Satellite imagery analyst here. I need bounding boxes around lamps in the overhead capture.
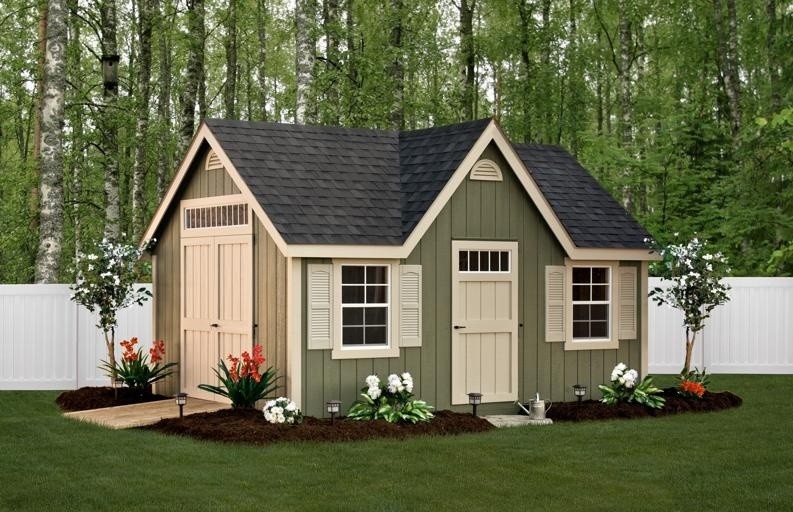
[467,384,586,415]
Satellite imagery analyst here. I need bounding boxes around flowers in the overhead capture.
[258,390,305,426]
[94,335,177,392]
[598,363,712,411]
[347,369,434,425]
[196,344,288,409]
